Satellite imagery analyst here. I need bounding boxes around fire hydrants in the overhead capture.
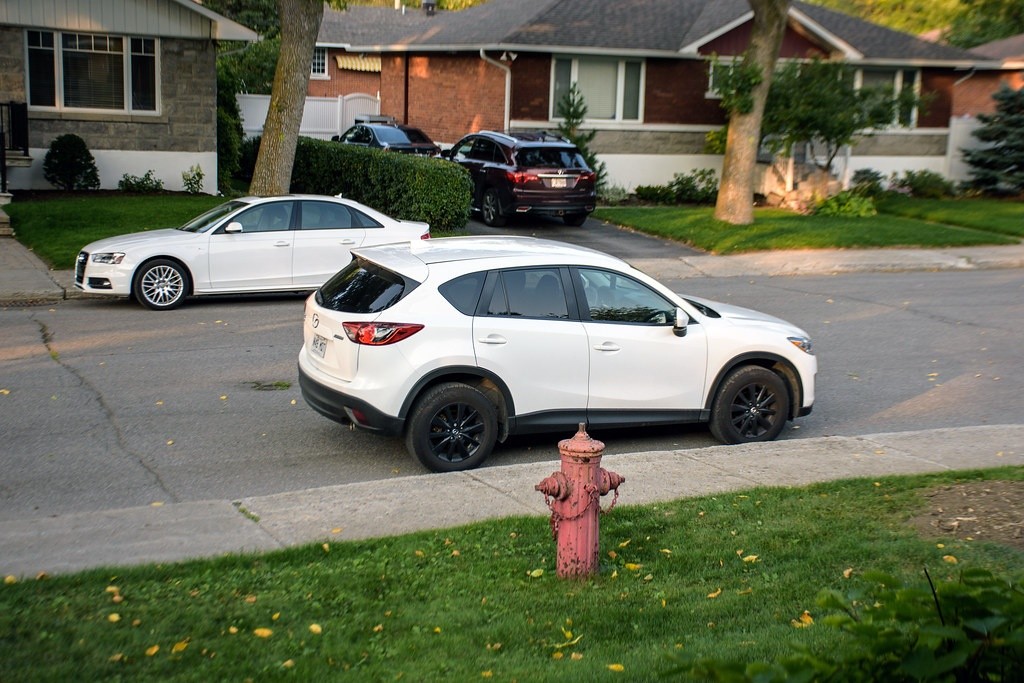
[533,420,628,585]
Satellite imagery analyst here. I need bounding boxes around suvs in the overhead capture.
[431,128,601,227]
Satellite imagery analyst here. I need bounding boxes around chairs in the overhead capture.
[264,206,288,229]
[535,275,563,316]
[504,271,534,317]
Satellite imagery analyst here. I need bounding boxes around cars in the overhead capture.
[297,234,820,476]
[329,123,441,161]
[72,193,432,312]
